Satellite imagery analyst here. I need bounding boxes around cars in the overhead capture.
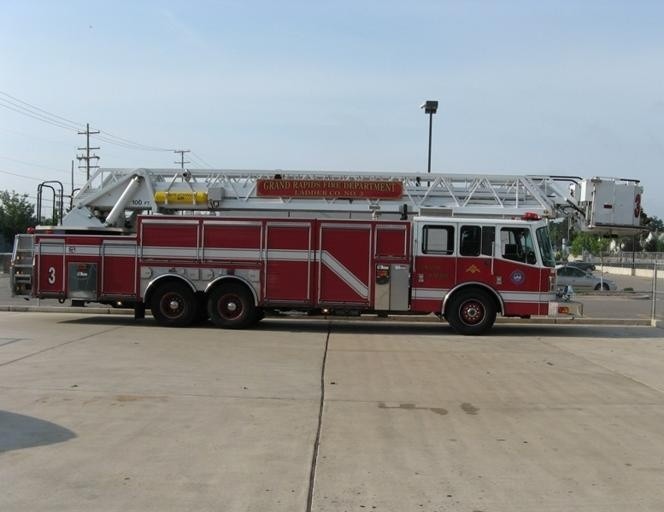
[554,260,616,291]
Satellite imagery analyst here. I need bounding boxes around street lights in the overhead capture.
[423,99,438,185]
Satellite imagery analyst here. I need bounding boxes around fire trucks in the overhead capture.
[10,168,651,336]
